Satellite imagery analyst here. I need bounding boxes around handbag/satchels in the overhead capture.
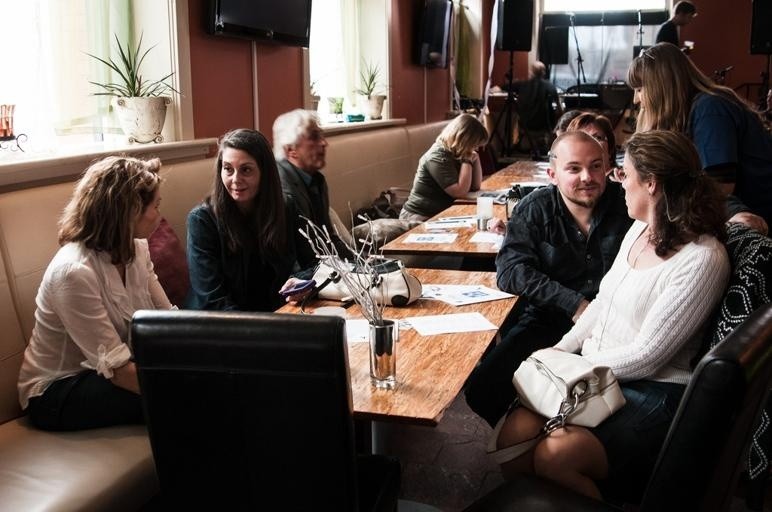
[301,256,422,317]
[484,346,626,464]
[506,184,547,221]
[356,190,398,222]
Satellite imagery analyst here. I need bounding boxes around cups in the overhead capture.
[476,197,494,230]
[507,198,520,220]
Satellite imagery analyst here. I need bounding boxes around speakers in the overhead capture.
[496,0,533,51]
[538,11,570,64]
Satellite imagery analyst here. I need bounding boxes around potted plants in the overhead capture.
[352,55,387,120]
[311,80,320,110]
[82,29,186,146]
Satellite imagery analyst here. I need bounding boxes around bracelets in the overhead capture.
[461,158,473,168]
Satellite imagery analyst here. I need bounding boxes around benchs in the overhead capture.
[0,123,451,511]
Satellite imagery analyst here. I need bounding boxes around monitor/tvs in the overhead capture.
[411,0,453,69]
[211,0,312,49]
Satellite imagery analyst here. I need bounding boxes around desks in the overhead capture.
[274,266,519,455]
[379,162,551,259]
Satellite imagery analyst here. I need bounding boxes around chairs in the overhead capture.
[131,309,402,512]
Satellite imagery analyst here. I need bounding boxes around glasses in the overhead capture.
[639,48,655,62]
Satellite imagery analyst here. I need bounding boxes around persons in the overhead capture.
[553,109,582,138]
[760,88,772,120]
[398,112,495,273]
[513,60,564,156]
[607,42,771,242]
[493,128,734,503]
[484,111,622,238]
[185,127,314,317]
[655,0,698,58]
[269,107,353,266]
[463,128,769,429]
[15,155,179,432]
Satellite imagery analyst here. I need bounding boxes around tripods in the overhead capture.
[483,51,542,160]
